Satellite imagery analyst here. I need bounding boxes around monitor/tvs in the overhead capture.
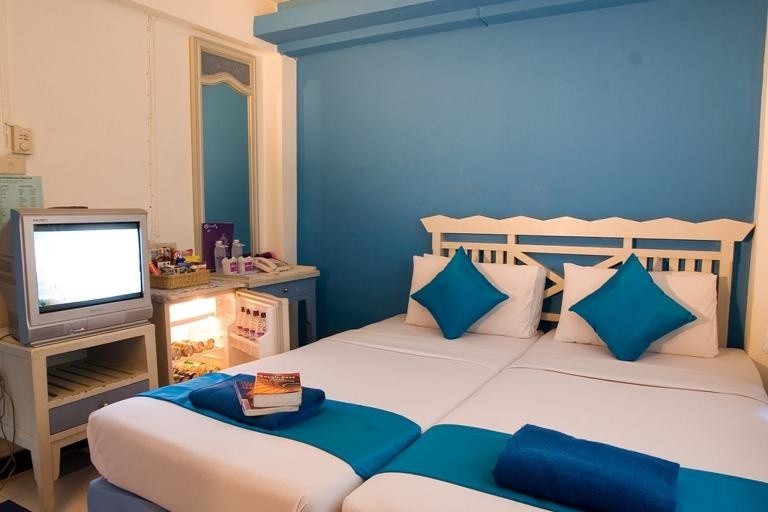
[0,206,154,347]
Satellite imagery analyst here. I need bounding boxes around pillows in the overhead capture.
[412,245,509,340]
[568,252,698,362]
[553,263,721,359]
[406,253,546,338]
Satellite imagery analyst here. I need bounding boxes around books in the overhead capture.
[234,380,300,416]
[254,371,303,406]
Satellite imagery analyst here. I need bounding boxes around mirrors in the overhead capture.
[190,37,260,255]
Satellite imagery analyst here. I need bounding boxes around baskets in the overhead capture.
[150,269,211,289]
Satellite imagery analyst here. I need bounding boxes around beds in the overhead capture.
[86,214,767,512]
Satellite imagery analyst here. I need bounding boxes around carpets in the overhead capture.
[0,500,32,512]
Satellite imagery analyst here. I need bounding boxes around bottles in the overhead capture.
[232,239,243,258]
[214,240,226,274]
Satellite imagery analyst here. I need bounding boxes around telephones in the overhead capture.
[254,257,290,273]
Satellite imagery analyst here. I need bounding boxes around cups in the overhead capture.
[238,256,254,274]
[222,257,237,275]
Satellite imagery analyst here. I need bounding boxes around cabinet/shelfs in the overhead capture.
[0,324,159,512]
[247,276,316,349]
